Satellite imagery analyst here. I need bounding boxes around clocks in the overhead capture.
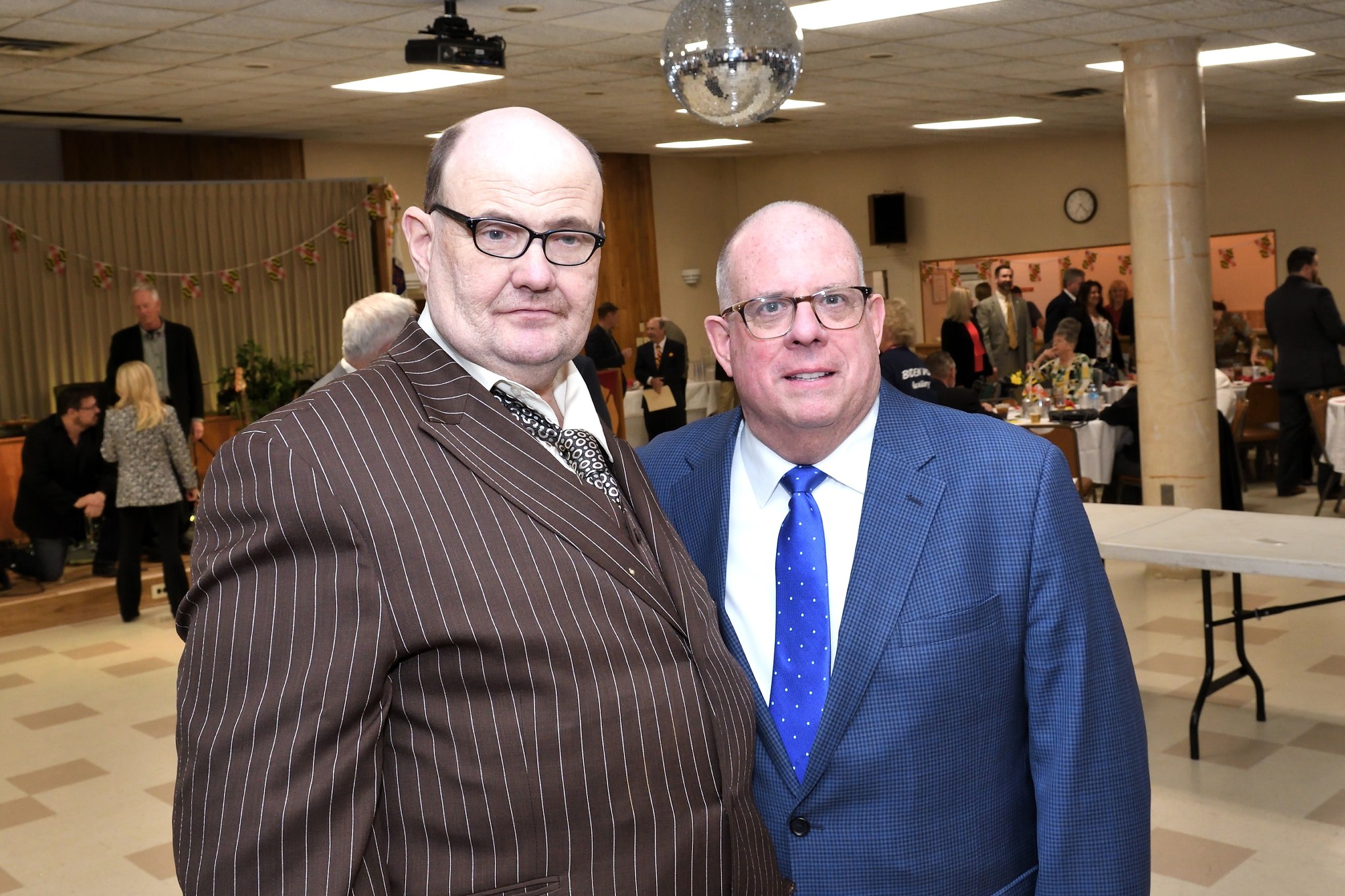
[1063,187,1098,224]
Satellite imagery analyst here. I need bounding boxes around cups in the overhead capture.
[1079,396,1092,409]
[995,403,1009,420]
[1053,386,1065,407]
[1029,406,1042,423]
[1233,363,1243,381]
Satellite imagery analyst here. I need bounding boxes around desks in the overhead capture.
[1100,379,1136,405]
[1230,378,1252,401]
[989,404,1129,500]
[1324,393,1345,511]
[1082,501,1192,570]
[622,379,722,450]
[1099,506,1345,762]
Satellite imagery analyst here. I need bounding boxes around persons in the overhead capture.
[1263,246,1345,500]
[101,362,197,623]
[585,302,627,400]
[1213,310,1260,366]
[878,298,938,404]
[940,265,1141,505]
[925,351,985,414]
[305,292,417,394]
[636,202,1152,896]
[572,355,612,431]
[715,359,740,414]
[0,387,116,590]
[105,289,205,563]
[172,105,794,896]
[633,317,687,443]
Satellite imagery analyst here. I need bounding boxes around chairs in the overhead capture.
[602,345,1345,517]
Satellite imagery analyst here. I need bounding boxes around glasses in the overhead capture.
[73,402,101,412]
[429,204,606,265]
[720,284,873,339]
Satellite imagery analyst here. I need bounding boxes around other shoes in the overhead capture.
[1278,485,1306,496]
[119,609,140,621]
[146,551,163,563]
[1301,476,1319,486]
[93,566,117,578]
[180,533,192,554]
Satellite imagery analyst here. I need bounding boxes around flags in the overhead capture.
[180,184,398,299]
[920,252,1132,287]
[1218,248,1236,269]
[1254,236,1276,258]
[6,224,156,289]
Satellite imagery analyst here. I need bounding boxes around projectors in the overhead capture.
[404,38,507,71]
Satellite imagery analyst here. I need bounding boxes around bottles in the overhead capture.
[1088,384,1097,409]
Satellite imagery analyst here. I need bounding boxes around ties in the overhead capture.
[488,386,624,515]
[767,466,831,788]
[656,345,662,368]
[1005,296,1019,349]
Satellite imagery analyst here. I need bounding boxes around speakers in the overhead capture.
[867,191,909,245]
